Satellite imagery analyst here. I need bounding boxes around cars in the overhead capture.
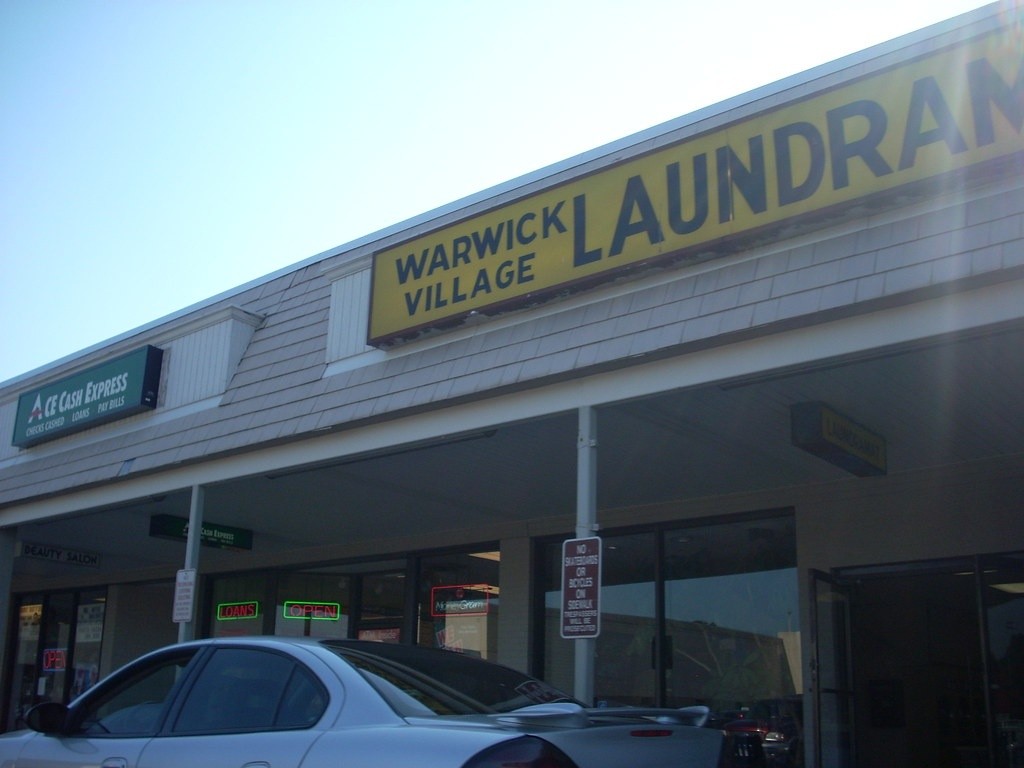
[0,636,728,768]
[709,698,798,768]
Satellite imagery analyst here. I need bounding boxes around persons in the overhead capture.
[69,664,99,704]
